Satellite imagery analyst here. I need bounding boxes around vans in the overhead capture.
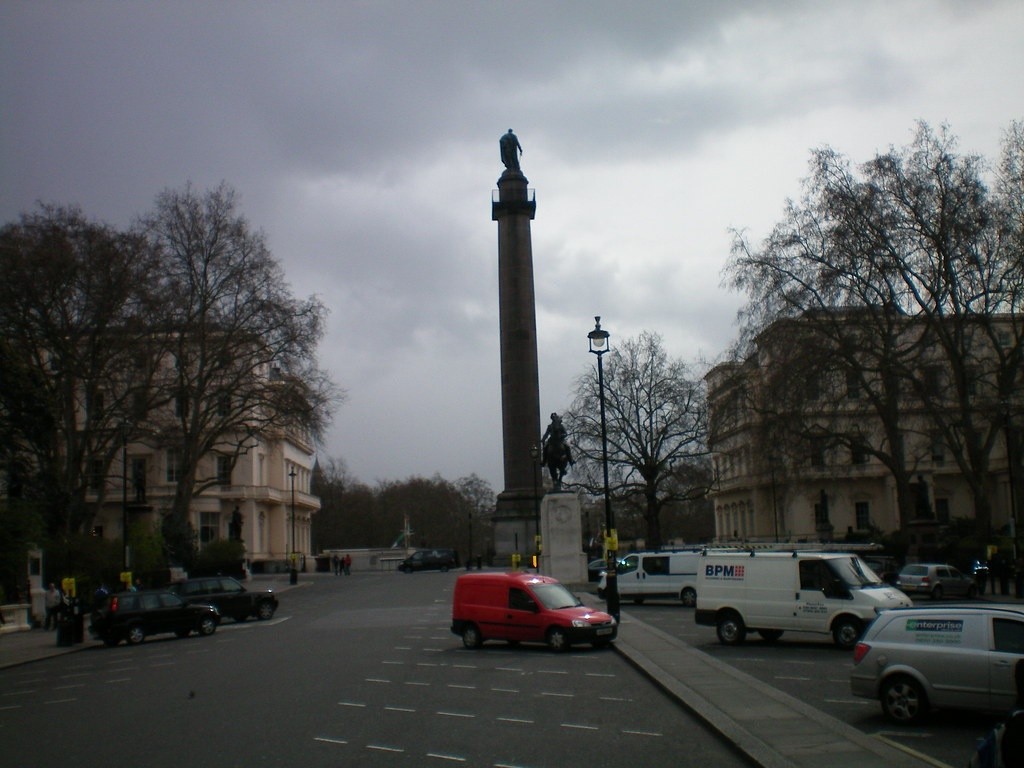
[598,549,699,607]
[397,548,460,574]
[695,547,912,648]
[450,570,617,652]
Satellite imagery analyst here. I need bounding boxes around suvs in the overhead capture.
[167,576,279,622]
[850,603,1024,724]
[89,589,221,646]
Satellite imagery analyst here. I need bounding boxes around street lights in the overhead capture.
[587,316,620,621]
[117,418,132,569]
[290,466,298,566]
[529,444,540,554]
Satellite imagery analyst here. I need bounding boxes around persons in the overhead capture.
[334,553,352,576]
[500,128,522,171]
[44,582,70,631]
[233,505,245,542]
[1018,558,1024,598]
[88,577,110,635]
[132,576,144,592]
[1000,658,1024,768]
[540,412,575,467]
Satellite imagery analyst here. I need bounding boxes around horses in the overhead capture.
[545,416,567,491]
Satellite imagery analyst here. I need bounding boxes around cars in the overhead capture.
[895,562,977,601]
[588,556,630,580]
